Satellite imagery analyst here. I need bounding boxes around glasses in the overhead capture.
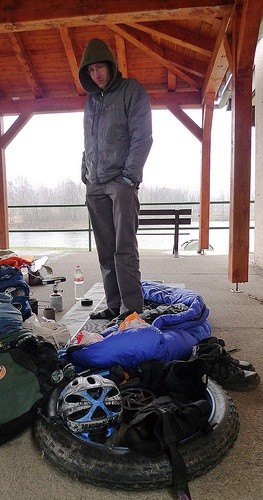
[86,65,104,75]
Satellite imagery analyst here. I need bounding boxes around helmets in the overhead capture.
[58,374,123,432]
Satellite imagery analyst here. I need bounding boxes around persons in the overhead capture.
[78,37,153,327]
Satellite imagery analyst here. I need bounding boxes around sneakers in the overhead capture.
[199,336,253,371]
[196,344,260,391]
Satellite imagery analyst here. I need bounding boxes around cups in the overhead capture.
[50,293,64,312]
[43,308,55,320]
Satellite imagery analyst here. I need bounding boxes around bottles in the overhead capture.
[73,265,85,300]
[21,263,29,284]
